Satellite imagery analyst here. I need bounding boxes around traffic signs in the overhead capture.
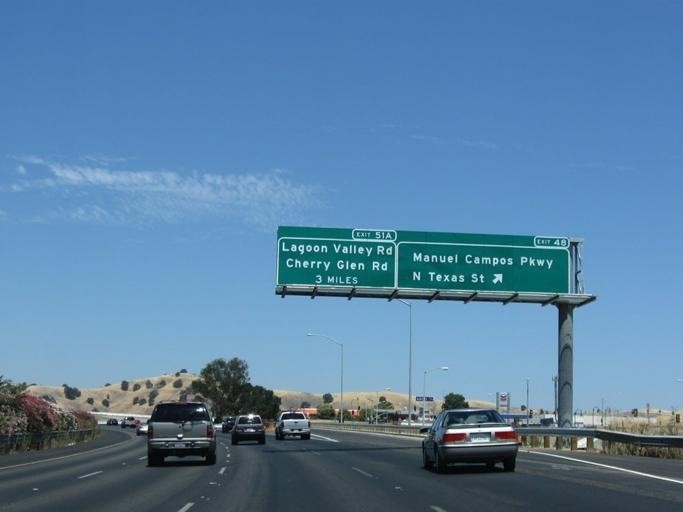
[274,225,575,297]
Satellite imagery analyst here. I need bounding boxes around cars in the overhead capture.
[136,423,148,435]
[418,405,520,473]
[106,418,117,425]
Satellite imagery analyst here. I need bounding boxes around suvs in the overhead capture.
[221,415,236,431]
[145,399,216,467]
[231,413,266,445]
[119,416,135,428]
[274,411,311,442]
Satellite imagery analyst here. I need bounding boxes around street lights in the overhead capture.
[306,332,344,424]
[591,406,600,428]
[423,366,450,425]
[375,387,391,424]
[574,407,584,427]
[551,372,559,421]
